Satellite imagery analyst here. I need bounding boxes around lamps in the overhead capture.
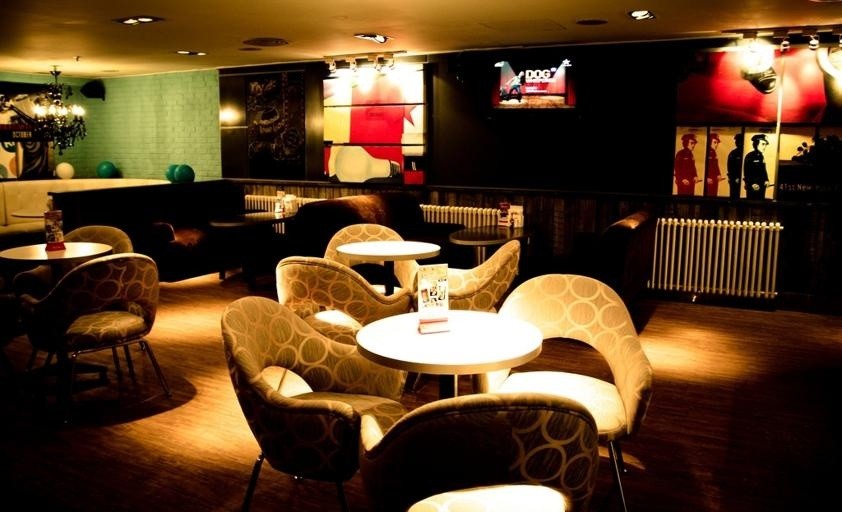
[31,66,87,154]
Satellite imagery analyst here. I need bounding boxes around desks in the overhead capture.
[336,241,441,296]
[356,310,542,400]
[208,210,296,292]
[449,226,528,265]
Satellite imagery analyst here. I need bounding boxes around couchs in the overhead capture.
[290,189,474,295]
[0,179,170,236]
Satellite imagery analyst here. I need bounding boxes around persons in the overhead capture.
[706,134,726,198]
[744,134,770,198]
[727,134,743,197]
[674,132,702,195]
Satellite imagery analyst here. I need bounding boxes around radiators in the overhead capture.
[245,195,324,234]
[418,204,502,265]
[647,218,784,298]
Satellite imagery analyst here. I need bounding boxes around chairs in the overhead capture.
[276,257,410,344]
[470,273,653,512]
[221,294,410,512]
[361,393,599,512]
[0,225,171,412]
[412,240,522,310]
[324,223,418,296]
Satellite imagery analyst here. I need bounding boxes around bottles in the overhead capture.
[275,197,282,213]
[498,203,511,226]
[421,289,429,303]
[45,209,66,252]
